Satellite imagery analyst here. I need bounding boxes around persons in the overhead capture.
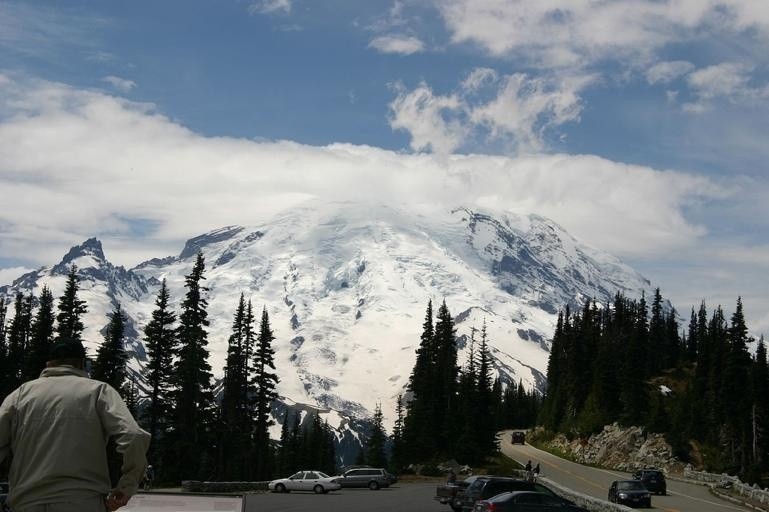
[446,468,456,484]
[143,465,155,490]
[533,464,540,483]
[1,338,150,512]
[525,461,532,483]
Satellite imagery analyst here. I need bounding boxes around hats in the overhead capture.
[47,337,86,367]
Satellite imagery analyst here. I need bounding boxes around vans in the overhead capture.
[341,467,390,491]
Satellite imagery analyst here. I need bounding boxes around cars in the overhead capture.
[267,469,341,493]
[511,432,526,445]
[437,471,588,512]
[609,479,651,508]
[634,467,666,496]
